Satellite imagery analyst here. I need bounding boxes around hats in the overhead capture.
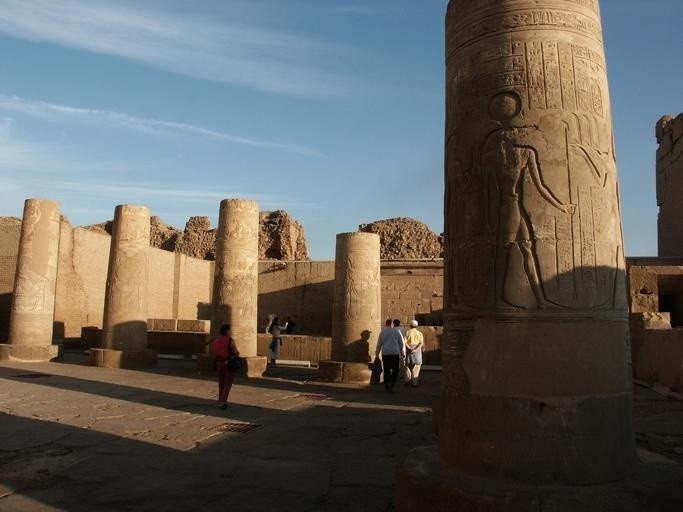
[411,319,418,327]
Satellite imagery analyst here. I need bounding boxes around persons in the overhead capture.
[375,319,404,394]
[392,319,406,380]
[213,324,239,409]
[403,320,424,386]
[267,318,289,368]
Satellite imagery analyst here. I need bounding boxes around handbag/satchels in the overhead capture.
[228,337,243,373]
